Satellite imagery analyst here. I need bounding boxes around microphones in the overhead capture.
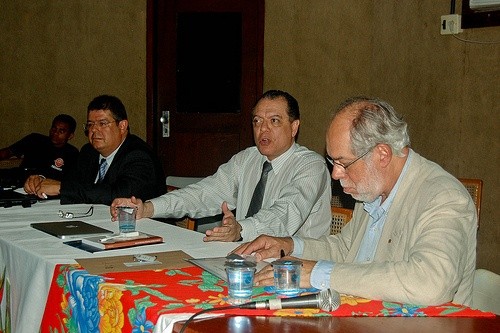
[239,288,344,312]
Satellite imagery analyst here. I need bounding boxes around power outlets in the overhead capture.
[440,13,463,36]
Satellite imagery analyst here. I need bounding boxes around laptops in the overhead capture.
[30,221,114,240]
[0,187,37,206]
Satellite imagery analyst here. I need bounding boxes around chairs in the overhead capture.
[472,268,500,315]
[459,176,484,233]
[166,183,196,230]
[330,204,354,237]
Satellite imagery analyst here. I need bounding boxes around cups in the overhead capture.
[116,206,137,234]
[224,261,257,305]
[271,260,302,295]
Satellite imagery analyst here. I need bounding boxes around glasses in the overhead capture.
[325,142,378,173]
[82,119,118,132]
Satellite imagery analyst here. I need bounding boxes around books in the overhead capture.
[82,230,163,249]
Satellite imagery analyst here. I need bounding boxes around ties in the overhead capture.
[98,158,107,184]
[245,160,273,220]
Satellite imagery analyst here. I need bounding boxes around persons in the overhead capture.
[110,90,334,242]
[25,94,177,225]
[228,95,477,307]
[0,114,79,182]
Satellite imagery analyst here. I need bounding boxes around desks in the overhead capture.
[0,199,500,333]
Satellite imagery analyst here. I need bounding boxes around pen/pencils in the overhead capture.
[41,192,48,199]
[280,249,285,258]
